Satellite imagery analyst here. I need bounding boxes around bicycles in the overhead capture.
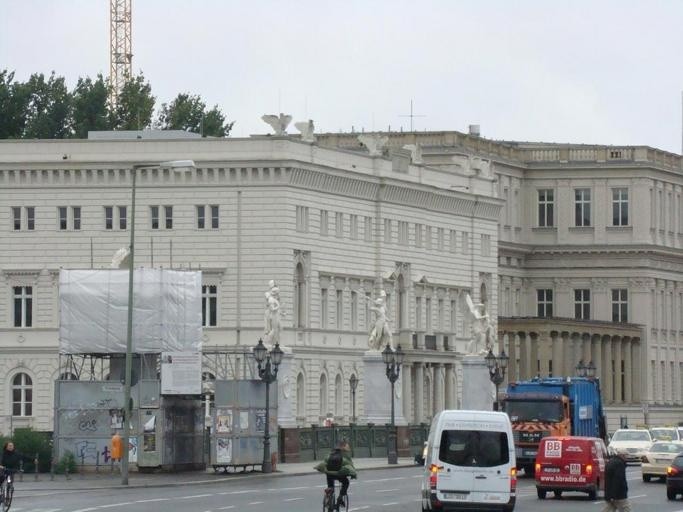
[0,465,25,512]
[321,473,355,512]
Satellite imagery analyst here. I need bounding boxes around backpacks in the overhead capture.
[327,449,342,471]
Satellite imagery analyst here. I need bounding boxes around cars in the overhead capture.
[414,441,428,466]
[605,426,683,501]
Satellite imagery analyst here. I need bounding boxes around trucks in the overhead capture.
[492,373,604,479]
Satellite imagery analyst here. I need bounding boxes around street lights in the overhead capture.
[120,160,200,484]
[574,359,595,378]
[484,346,510,412]
[250,339,283,471]
[379,341,403,463]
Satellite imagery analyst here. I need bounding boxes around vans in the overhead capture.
[419,410,516,512]
[532,433,608,501]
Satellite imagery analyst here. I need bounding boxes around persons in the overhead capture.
[264,279,280,336]
[312,441,358,508]
[485,319,495,352]
[472,303,489,341]
[370,298,396,352]
[0,441,39,487]
[267,287,286,347]
[601,447,633,512]
